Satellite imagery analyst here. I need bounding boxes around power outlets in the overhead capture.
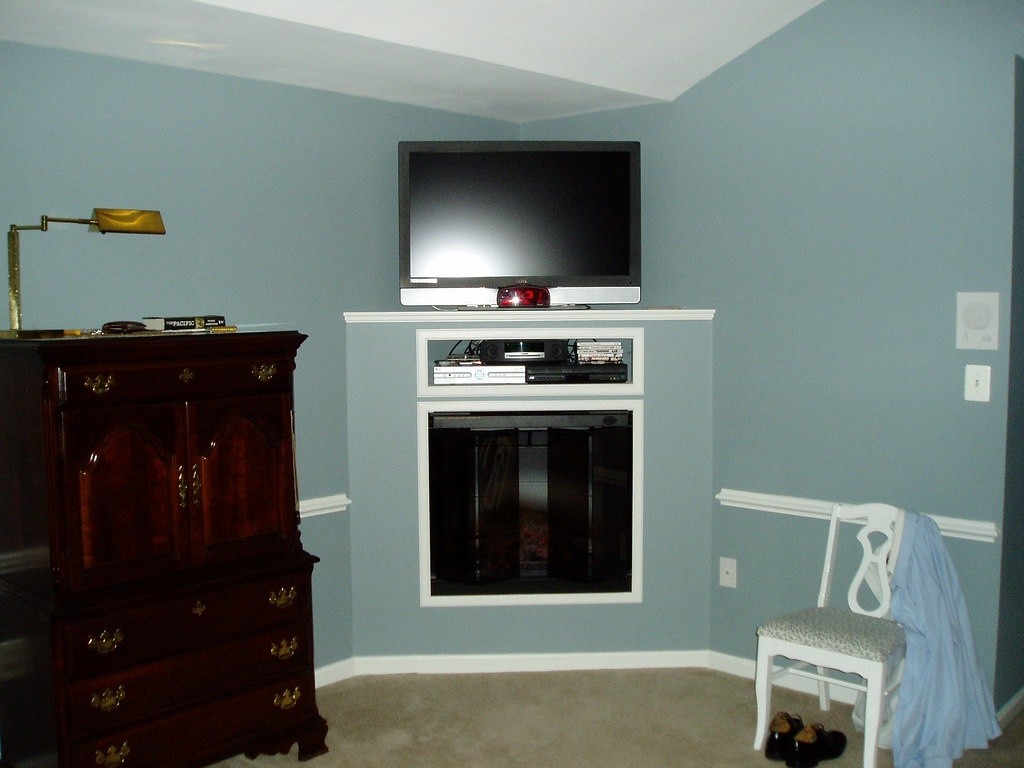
[720,557,736,588]
[964,364,993,400]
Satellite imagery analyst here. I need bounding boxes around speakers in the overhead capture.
[480,341,504,365]
[544,340,568,361]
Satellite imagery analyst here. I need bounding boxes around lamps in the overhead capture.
[7,209,167,332]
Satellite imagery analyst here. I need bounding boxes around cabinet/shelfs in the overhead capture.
[0,330,329,768]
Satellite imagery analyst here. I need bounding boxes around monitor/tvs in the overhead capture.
[398,141,642,313]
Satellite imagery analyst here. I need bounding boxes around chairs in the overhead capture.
[753,503,924,768]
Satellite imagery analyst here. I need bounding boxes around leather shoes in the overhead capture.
[786,723,847,768]
[765,712,804,761]
